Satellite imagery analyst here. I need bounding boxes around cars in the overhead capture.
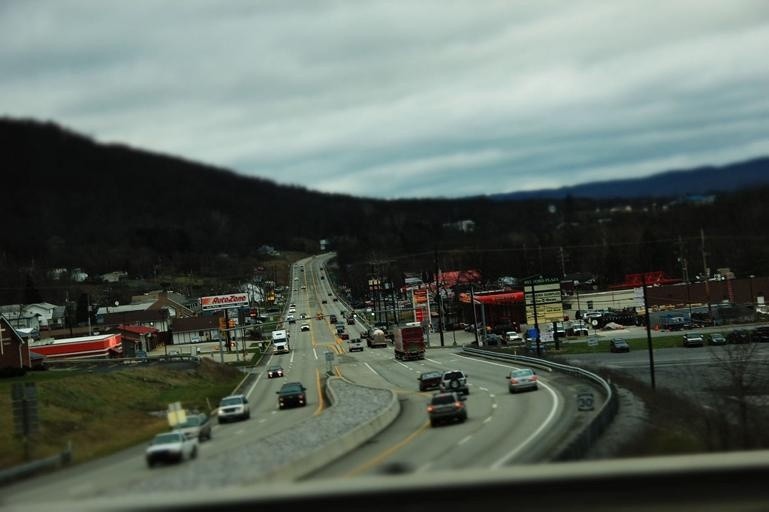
[275,381,307,409]
[486,322,588,342]
[575,310,614,320]
[191,336,202,343]
[213,334,220,341]
[267,366,283,378]
[682,326,769,349]
[145,412,212,470]
[505,368,538,392]
[330,315,367,352]
[321,291,338,304]
[417,369,469,428]
[287,303,310,331]
[293,286,306,291]
[135,350,147,362]
[610,336,631,353]
[218,394,249,423]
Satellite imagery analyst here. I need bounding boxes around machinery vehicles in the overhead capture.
[367,328,388,348]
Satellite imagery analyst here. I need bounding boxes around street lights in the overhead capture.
[369,281,380,320]
[573,279,581,310]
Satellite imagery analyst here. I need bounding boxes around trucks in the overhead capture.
[271,330,290,354]
[394,326,425,362]
